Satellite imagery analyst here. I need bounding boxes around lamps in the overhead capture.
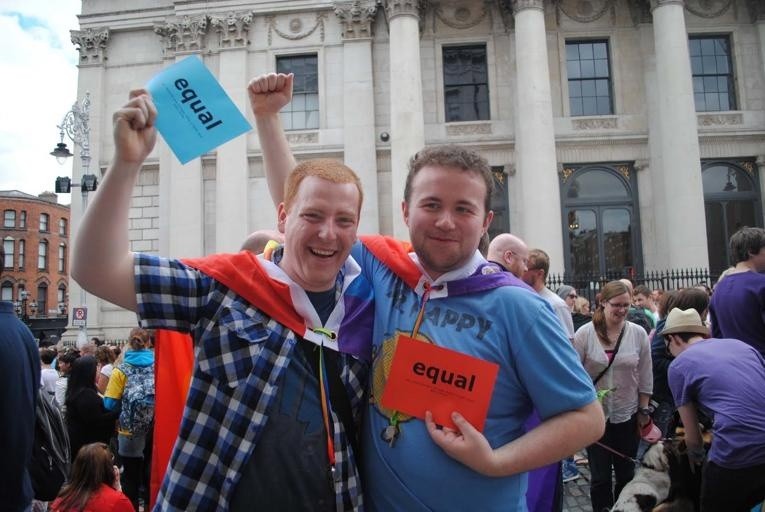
[49,95,93,168]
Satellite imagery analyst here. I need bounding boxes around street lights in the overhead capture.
[49,88,100,354]
[13,288,38,326]
[58,291,69,327]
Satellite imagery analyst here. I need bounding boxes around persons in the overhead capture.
[487,227,765,511]
[0,245,41,512]
[40,328,155,512]
[248,72,606,512]
[69,87,369,512]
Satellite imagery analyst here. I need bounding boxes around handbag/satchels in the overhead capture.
[30,385,71,502]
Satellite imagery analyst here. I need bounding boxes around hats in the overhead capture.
[658,307,710,339]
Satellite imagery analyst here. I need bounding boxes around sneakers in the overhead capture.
[561,460,580,482]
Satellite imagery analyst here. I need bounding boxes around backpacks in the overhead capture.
[112,362,155,438]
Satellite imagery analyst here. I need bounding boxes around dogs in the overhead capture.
[607,438,681,512]
[675,422,714,462]
